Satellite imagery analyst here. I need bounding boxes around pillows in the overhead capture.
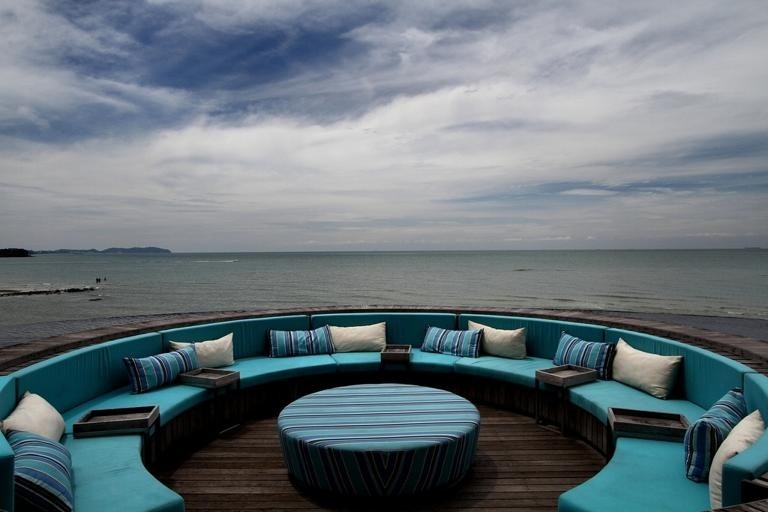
[678,386,765,512]
[1,389,77,509]
